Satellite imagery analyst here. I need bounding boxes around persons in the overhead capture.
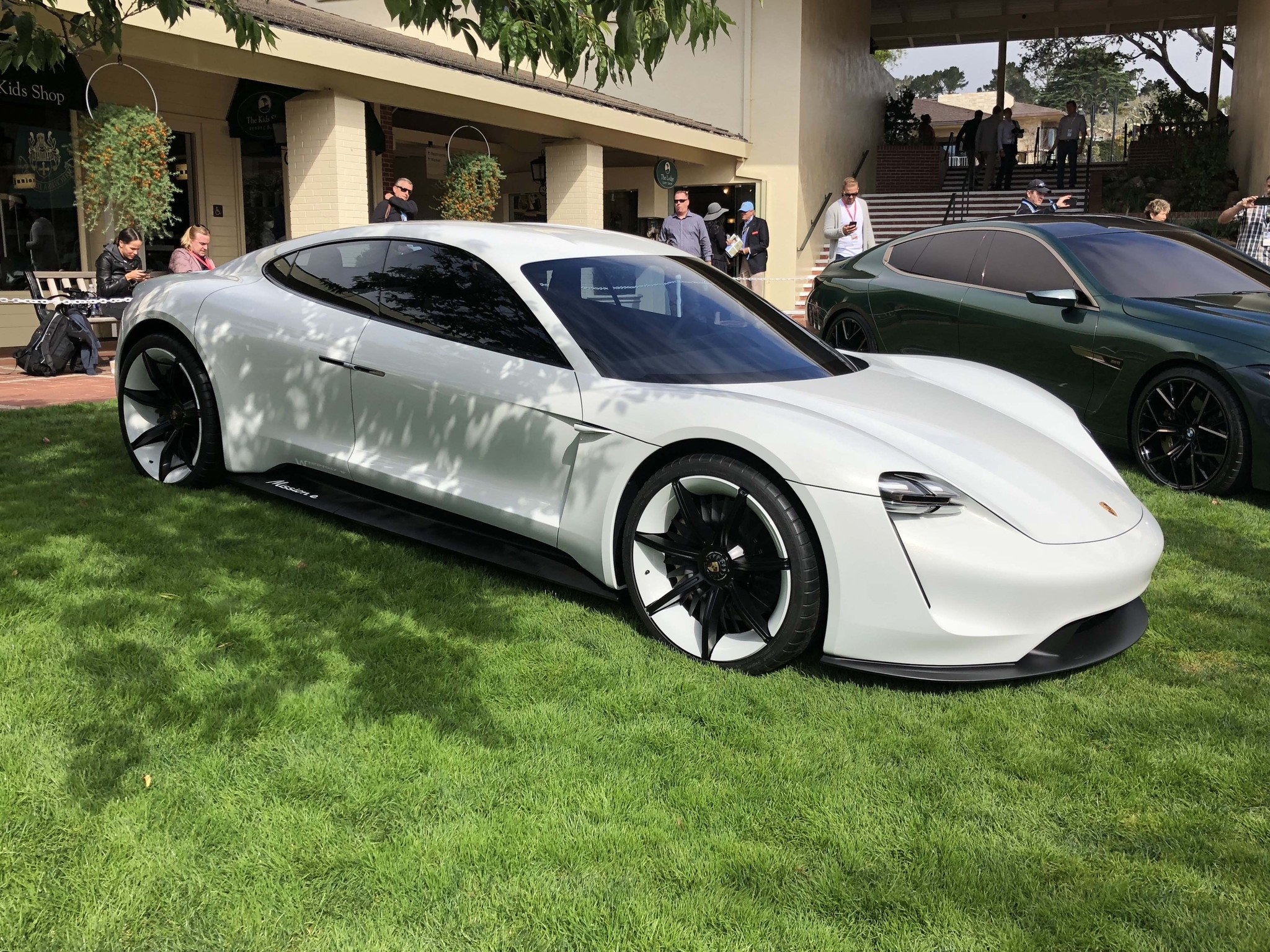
[704,203,733,276]
[955,105,1024,191]
[657,190,714,266]
[167,223,216,274]
[733,201,769,298]
[258,215,279,250]
[1047,100,1087,189]
[94,226,151,320]
[918,114,937,142]
[1217,174,1270,267]
[1014,179,1072,215]
[370,177,419,223]
[822,177,876,263]
[21,204,57,271]
[1144,199,1171,222]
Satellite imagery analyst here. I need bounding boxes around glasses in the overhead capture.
[395,185,413,195]
[1030,183,1046,188]
[189,224,205,231]
[674,199,687,203]
[843,189,858,196]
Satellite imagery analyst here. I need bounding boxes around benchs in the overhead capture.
[24,271,118,357]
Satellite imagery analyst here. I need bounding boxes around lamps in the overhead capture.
[272,122,288,164]
[530,149,546,197]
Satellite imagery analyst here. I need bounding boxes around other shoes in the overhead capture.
[1055,186,1063,189]
[1069,184,1075,188]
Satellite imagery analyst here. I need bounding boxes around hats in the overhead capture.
[703,202,729,221]
[738,201,753,212]
[1027,179,1052,193]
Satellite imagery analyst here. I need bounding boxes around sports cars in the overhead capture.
[111,222,1167,687]
[804,214,1270,494]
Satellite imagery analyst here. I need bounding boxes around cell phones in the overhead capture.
[1066,197,1078,205]
[1254,197,1270,205]
[849,221,857,233]
[141,269,153,275]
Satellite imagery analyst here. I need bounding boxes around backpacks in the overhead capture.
[12,311,76,376]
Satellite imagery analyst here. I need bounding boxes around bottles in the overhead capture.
[87,284,100,318]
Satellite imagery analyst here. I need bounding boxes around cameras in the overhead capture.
[1012,127,1024,134]
